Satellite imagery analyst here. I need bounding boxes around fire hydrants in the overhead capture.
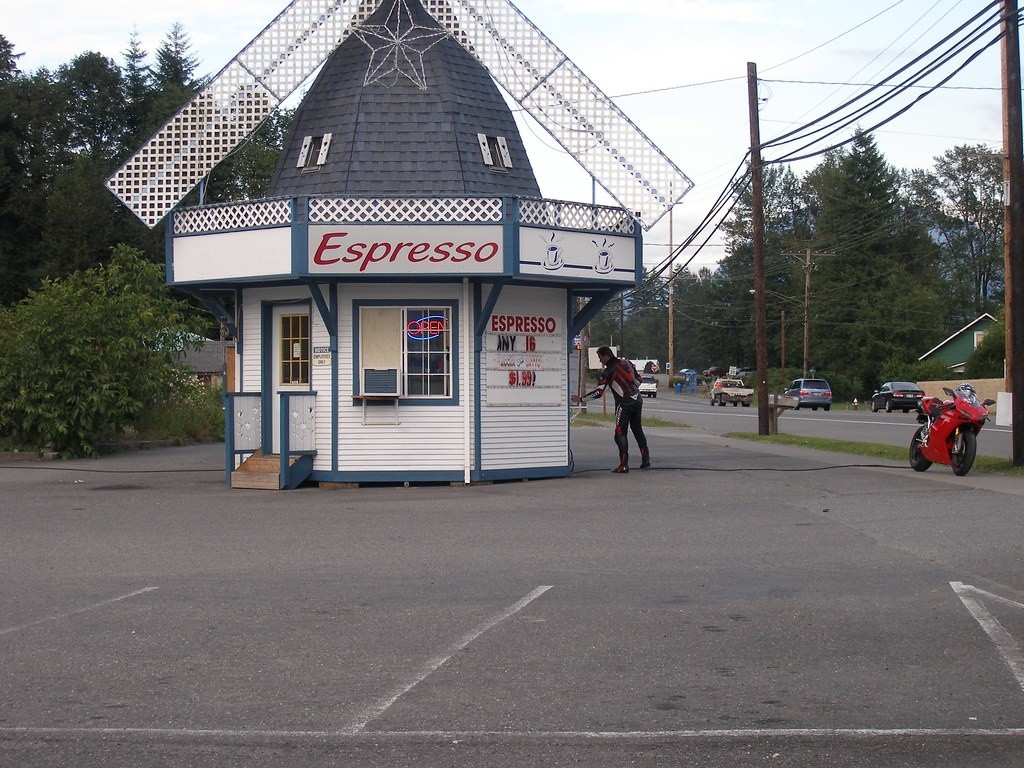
[852,398,859,410]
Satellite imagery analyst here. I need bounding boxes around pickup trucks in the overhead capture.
[708,378,754,407]
[638,374,659,398]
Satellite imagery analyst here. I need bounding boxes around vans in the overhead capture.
[782,377,832,412]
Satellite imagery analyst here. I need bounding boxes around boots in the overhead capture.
[612,452,629,472]
[639,447,650,468]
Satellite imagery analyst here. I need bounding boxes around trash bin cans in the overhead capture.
[675,382,683,393]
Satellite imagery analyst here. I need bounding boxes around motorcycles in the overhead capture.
[908,387,996,478]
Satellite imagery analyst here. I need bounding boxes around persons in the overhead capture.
[570,346,651,473]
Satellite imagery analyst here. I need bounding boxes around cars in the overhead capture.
[703,366,726,378]
[735,367,756,376]
[869,381,925,413]
